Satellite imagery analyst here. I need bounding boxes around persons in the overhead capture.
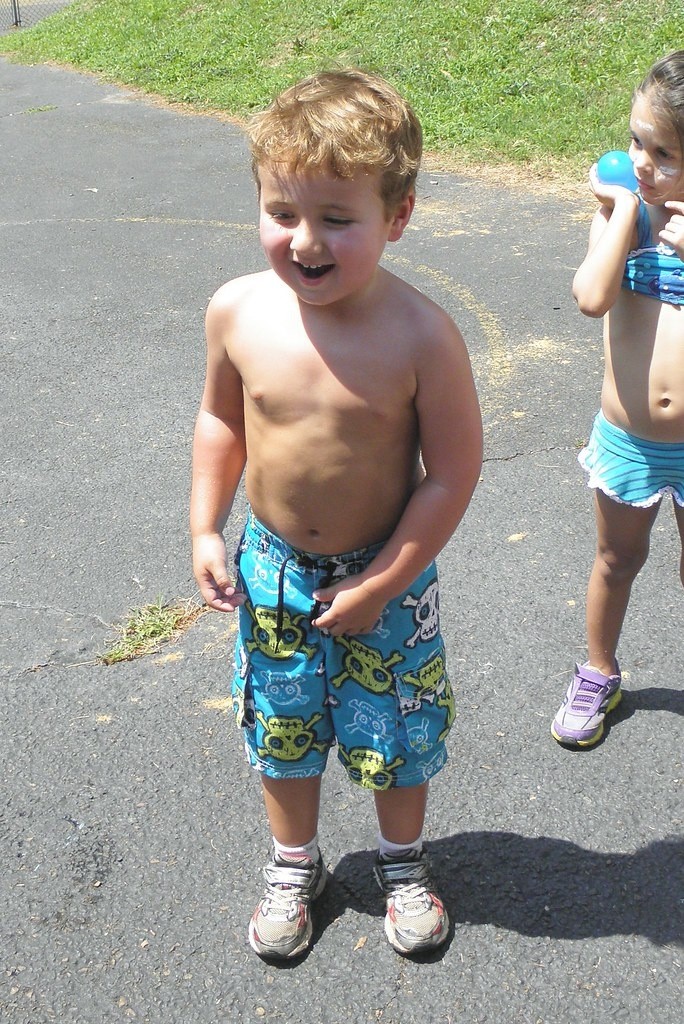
[186,72,482,962]
[550,53,684,750]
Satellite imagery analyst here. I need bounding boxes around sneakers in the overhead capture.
[248,846,327,959]
[373,845,449,953]
[550,656,621,747]
